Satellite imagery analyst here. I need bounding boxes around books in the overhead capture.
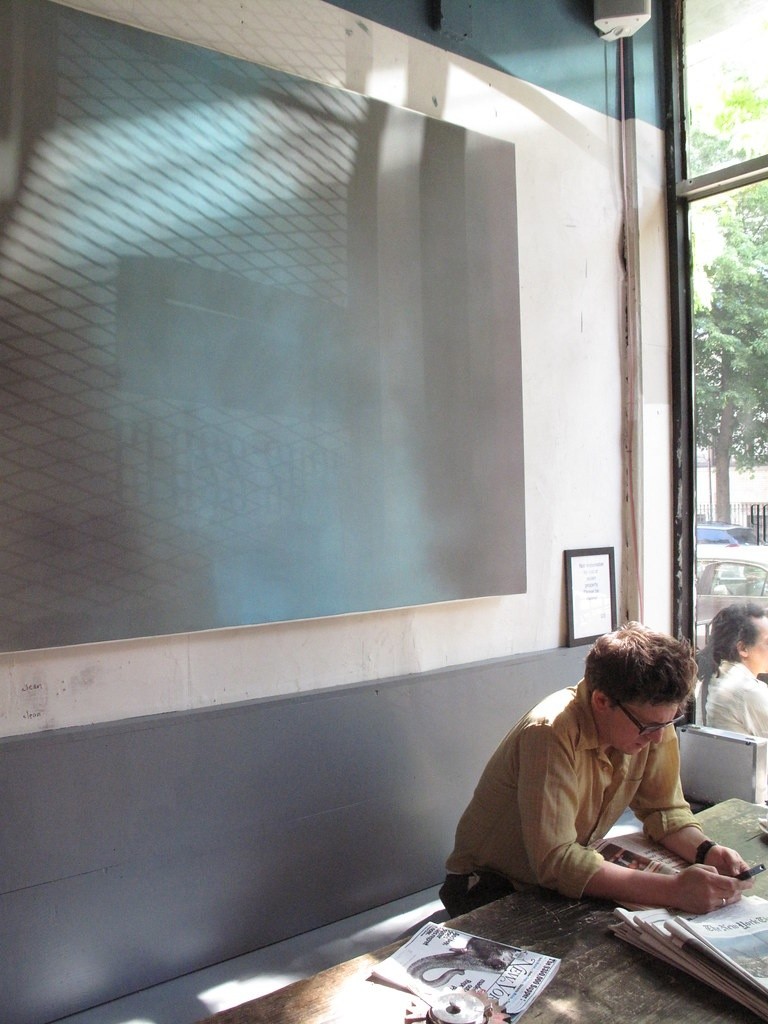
[370,921,562,1023]
[579,829,696,911]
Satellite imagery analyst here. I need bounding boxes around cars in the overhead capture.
[696,522,764,546]
[695,543,768,621]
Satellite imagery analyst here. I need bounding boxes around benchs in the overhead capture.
[0,644,768,1024]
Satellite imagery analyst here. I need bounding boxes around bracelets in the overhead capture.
[694,839,717,864]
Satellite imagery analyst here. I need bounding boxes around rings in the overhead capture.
[721,898,727,908]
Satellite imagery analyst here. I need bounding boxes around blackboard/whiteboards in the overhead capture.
[0,1,532,654]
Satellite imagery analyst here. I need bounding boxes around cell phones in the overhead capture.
[736,863,766,880]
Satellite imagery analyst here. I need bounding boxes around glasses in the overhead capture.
[599,687,685,736]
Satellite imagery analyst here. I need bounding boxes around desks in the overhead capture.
[191,797,768,1024]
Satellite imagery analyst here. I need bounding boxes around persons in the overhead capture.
[694,602,767,740]
[439,620,757,918]
